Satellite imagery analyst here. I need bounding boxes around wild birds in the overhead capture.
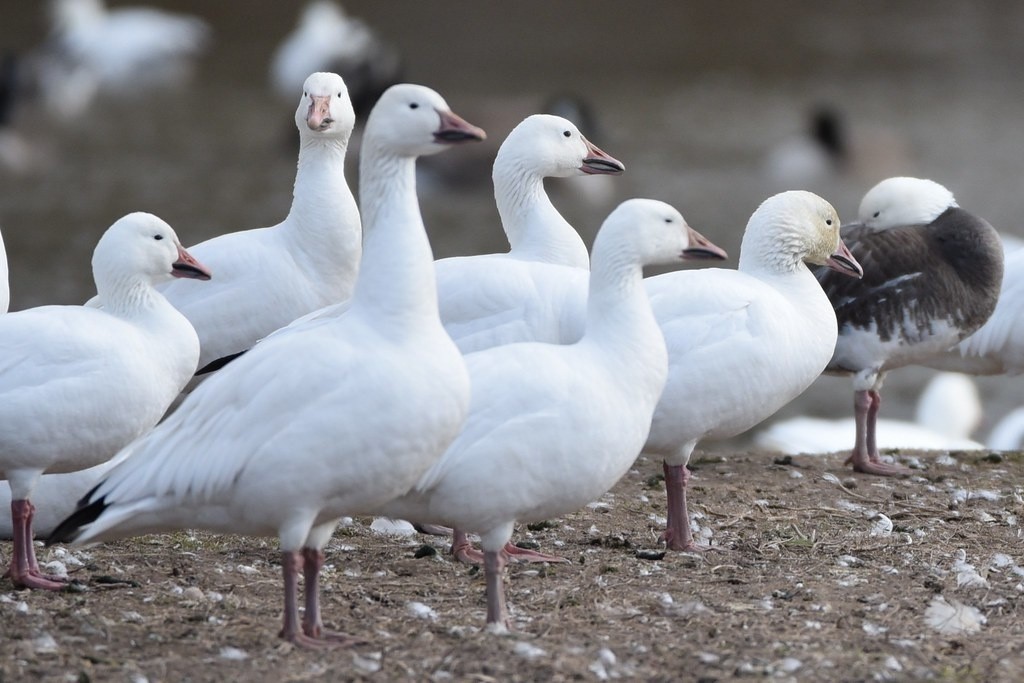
[634,188,867,555]
[43,80,488,655]
[419,114,627,572]
[2,209,213,595]
[356,198,733,642]
[796,173,1006,478]
[81,71,365,376]
